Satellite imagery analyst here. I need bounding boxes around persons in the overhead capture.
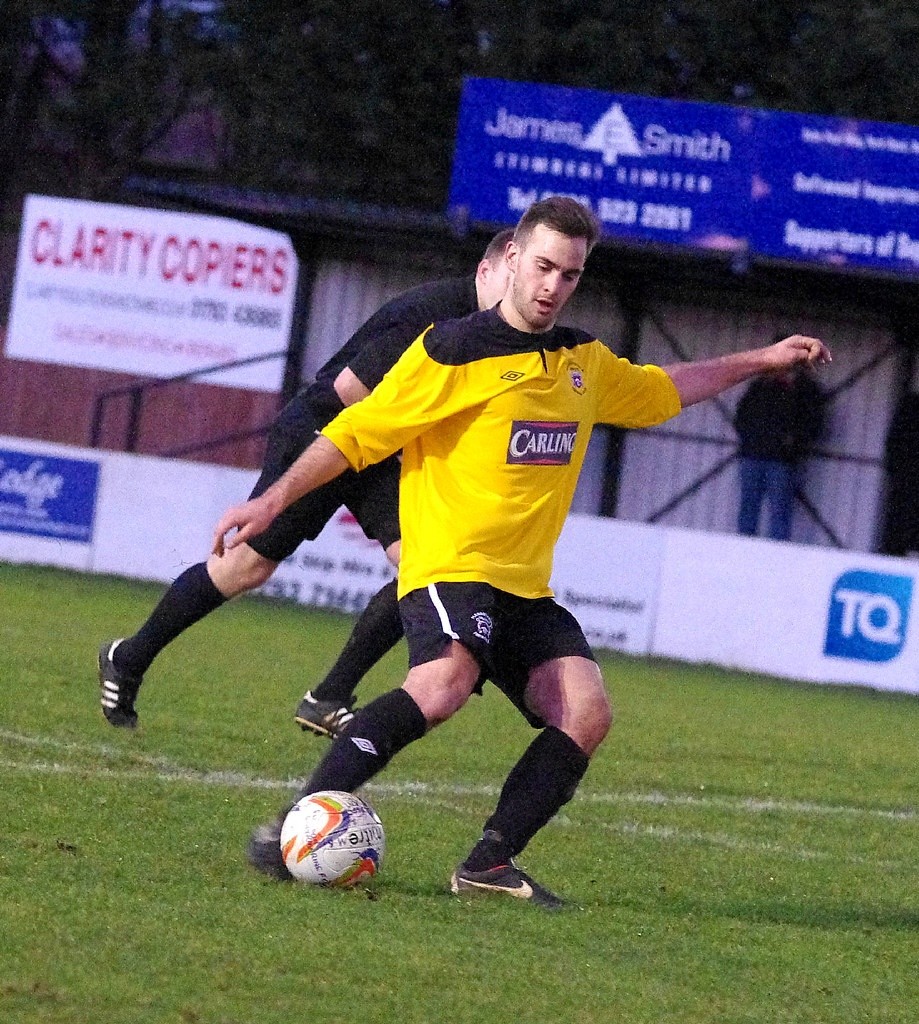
[734,326,825,541]
[213,198,832,905]
[99,225,518,730]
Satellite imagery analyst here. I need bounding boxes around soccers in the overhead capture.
[279,790,386,888]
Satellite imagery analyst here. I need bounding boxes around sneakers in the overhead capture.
[450,855,579,916]
[293,689,363,741]
[243,818,296,882]
[97,637,143,730]
